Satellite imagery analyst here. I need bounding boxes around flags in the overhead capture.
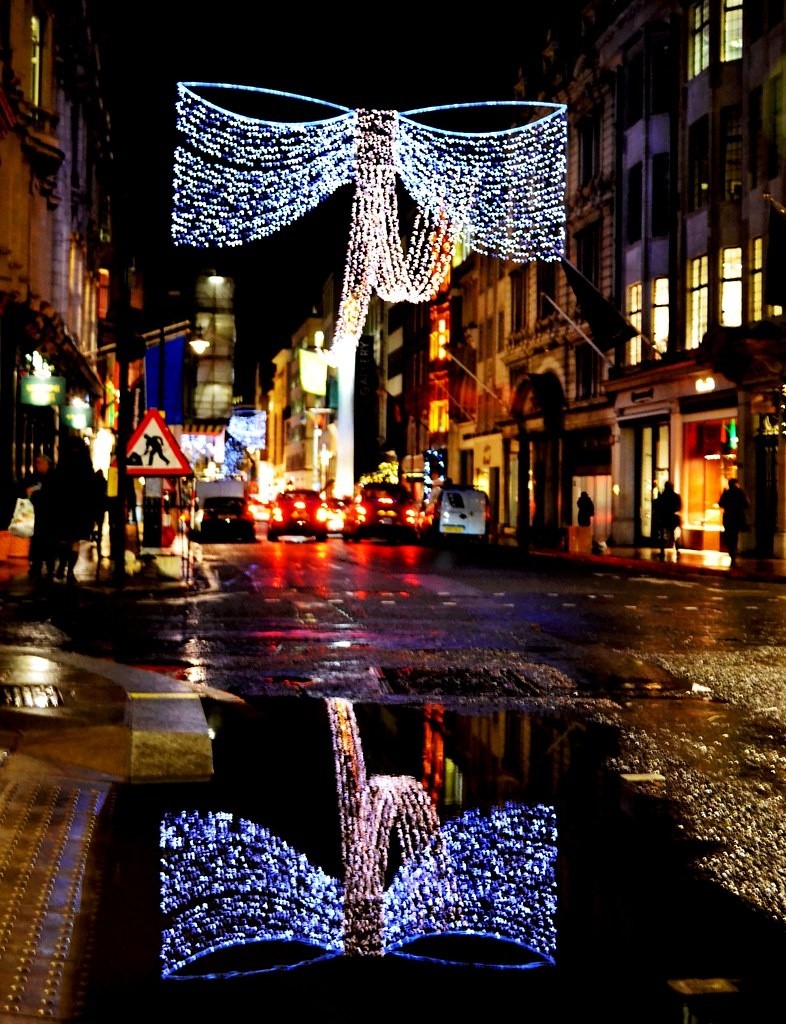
[764,197,785,305]
[557,253,639,353]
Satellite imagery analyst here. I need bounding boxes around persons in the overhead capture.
[27,434,99,576]
[719,478,752,561]
[576,491,594,526]
[655,482,683,557]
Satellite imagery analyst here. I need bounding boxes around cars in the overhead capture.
[188,479,499,548]
